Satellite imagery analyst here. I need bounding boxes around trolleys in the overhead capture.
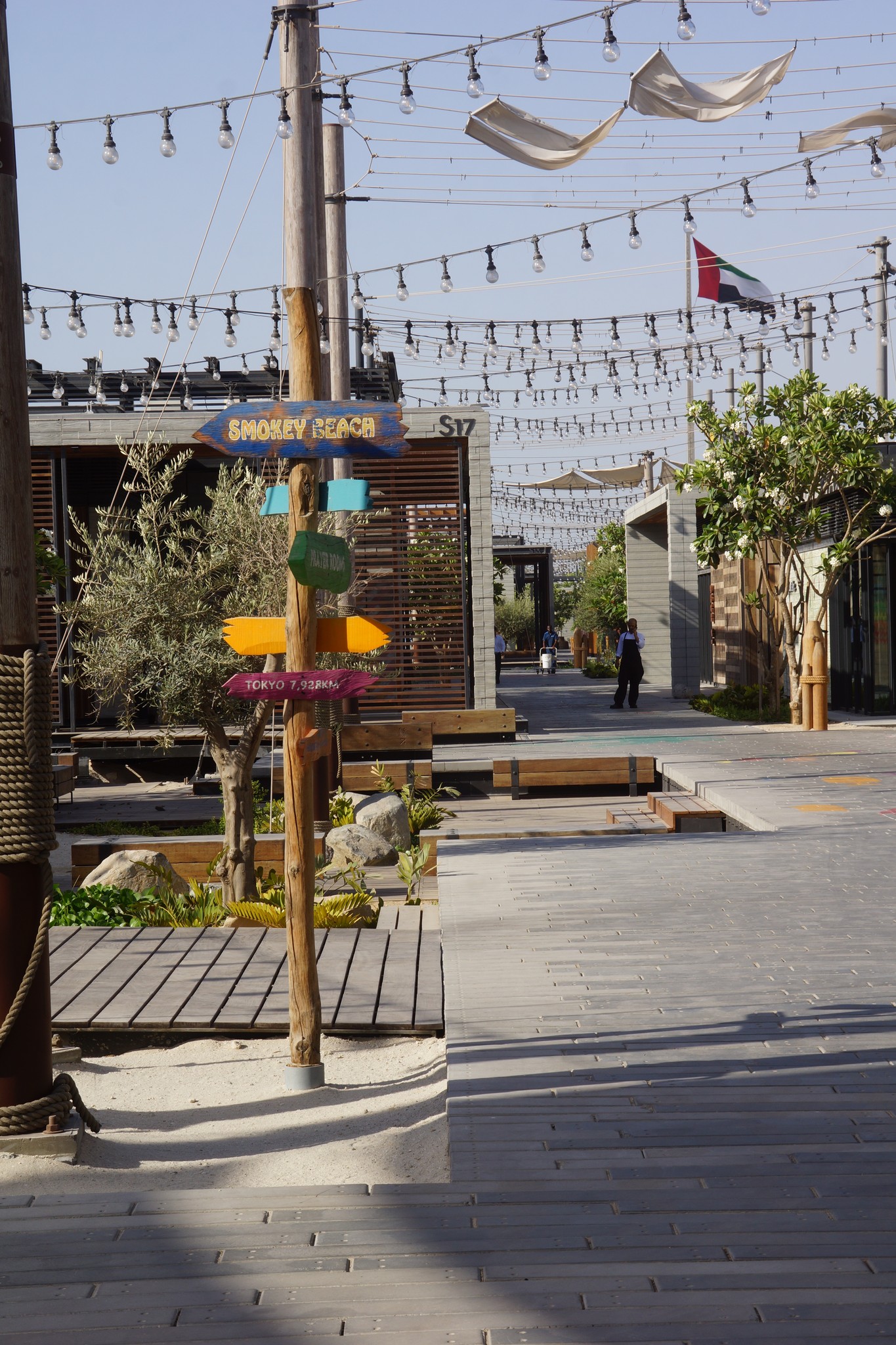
[533,647,557,674]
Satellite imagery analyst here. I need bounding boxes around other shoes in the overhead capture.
[496,678,500,684]
[629,704,638,709]
[610,703,624,709]
[551,670,556,673]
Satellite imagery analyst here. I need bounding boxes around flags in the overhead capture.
[692,236,777,323]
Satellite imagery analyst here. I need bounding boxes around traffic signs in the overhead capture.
[286,531,354,594]
[192,401,413,460]
[219,669,381,700]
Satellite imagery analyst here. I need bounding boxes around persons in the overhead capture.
[542,625,559,672]
[610,618,645,708]
[494,626,506,684]
